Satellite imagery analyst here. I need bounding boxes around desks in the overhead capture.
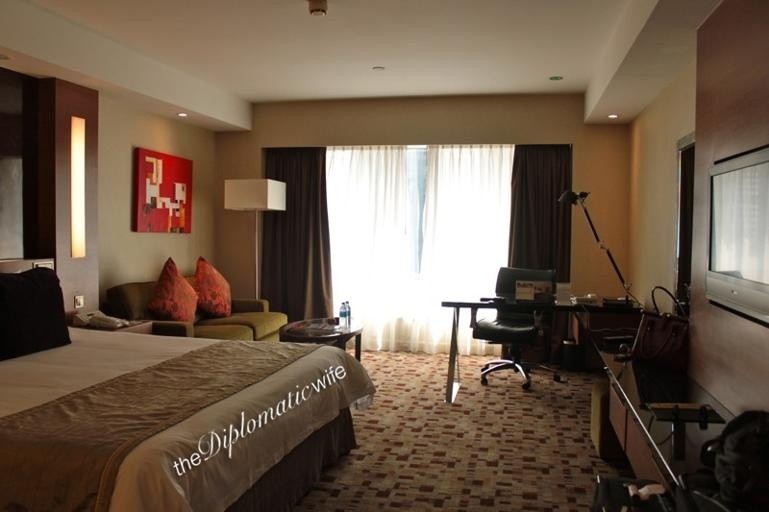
[442,297,644,404]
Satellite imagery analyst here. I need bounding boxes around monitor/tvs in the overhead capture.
[705,147,769,325]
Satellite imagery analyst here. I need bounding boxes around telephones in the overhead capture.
[88,315,130,328]
[570,293,598,305]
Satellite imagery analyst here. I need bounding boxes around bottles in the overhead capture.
[671,435,685,462]
[339,301,352,328]
[668,405,685,434]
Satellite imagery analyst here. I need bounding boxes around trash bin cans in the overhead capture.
[563,339,584,373]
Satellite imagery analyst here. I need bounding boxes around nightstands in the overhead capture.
[68,321,153,334]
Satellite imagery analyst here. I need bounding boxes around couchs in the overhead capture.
[106,274,287,341]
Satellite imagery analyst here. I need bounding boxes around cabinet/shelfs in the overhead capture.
[583,328,737,502]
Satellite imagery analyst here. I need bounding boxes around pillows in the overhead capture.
[0,267,71,362]
[148,257,199,326]
[193,256,232,317]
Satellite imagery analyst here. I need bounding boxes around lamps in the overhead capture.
[558,190,631,301]
[224,179,286,300]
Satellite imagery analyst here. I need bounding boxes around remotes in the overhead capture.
[480,297,504,301]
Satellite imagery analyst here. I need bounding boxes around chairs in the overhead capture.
[469,267,561,389]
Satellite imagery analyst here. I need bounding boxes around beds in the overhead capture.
[0,259,375,512]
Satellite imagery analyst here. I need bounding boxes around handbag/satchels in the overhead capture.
[628,285,691,370]
